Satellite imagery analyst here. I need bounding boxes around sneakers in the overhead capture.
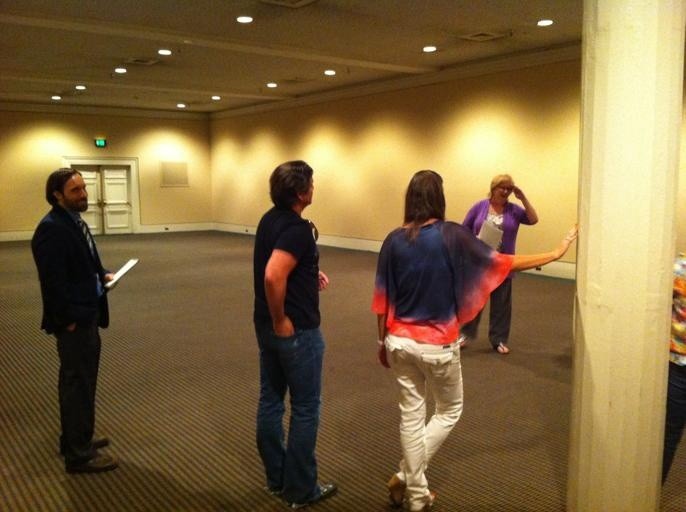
[387,472,402,505]
[288,484,335,508]
[426,490,438,498]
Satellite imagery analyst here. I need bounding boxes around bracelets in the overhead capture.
[376,336,385,346]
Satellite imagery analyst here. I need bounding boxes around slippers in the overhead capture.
[498,343,509,354]
[456,336,468,347]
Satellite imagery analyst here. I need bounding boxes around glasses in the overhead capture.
[498,186,513,193]
[305,220,318,241]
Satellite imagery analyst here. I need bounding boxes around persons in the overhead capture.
[660,250,686,489]
[371,170,578,512]
[252,161,338,508]
[459,172,538,355]
[29,170,119,473]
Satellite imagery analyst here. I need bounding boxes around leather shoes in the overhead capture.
[67,455,117,472]
[89,435,108,449]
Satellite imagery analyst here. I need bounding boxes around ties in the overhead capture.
[77,217,94,257]
